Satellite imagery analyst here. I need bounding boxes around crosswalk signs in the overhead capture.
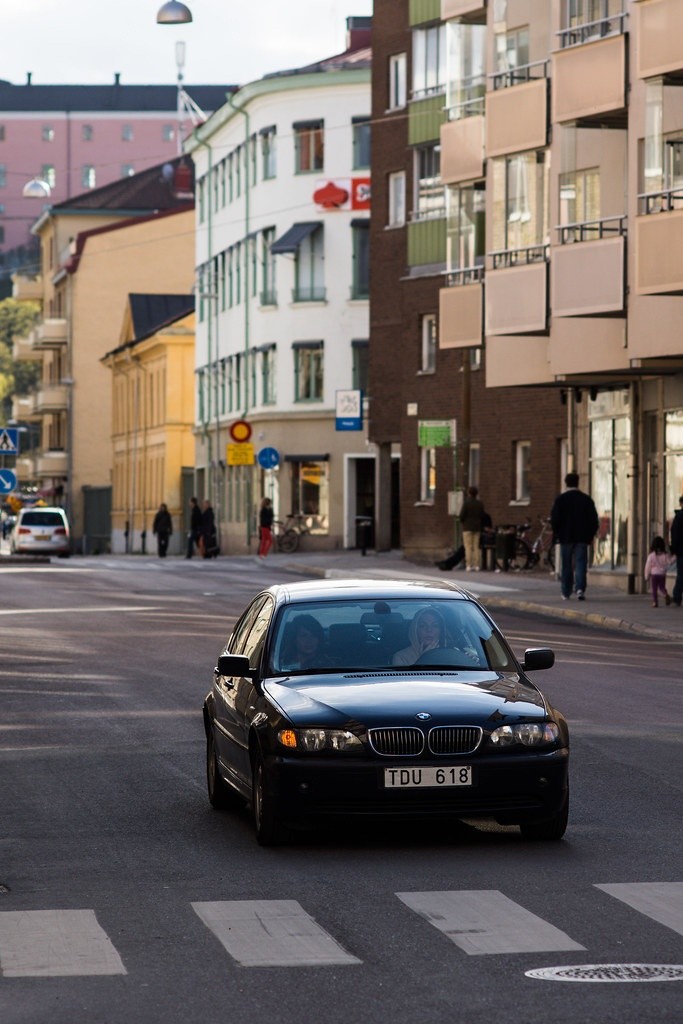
[0,427,20,456]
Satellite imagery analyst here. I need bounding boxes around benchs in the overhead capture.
[328,623,401,665]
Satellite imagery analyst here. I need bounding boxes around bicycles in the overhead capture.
[268,514,299,554]
[527,518,557,571]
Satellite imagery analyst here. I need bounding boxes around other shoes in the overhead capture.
[466,566,481,572]
[665,596,671,605]
[653,601,658,607]
[434,561,452,571]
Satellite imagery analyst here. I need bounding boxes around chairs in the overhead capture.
[398,618,413,651]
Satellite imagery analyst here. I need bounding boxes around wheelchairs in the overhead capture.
[489,520,531,572]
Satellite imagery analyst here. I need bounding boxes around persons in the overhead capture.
[153,503,173,559]
[434,486,492,572]
[279,614,349,670]
[393,606,480,666]
[185,497,220,560]
[259,498,274,559]
[550,473,599,601]
[668,494,683,608]
[644,536,677,608]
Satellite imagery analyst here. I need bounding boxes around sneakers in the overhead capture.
[561,594,569,600]
[576,590,586,600]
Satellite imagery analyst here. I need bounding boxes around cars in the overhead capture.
[8,506,71,559]
[202,579,571,843]
